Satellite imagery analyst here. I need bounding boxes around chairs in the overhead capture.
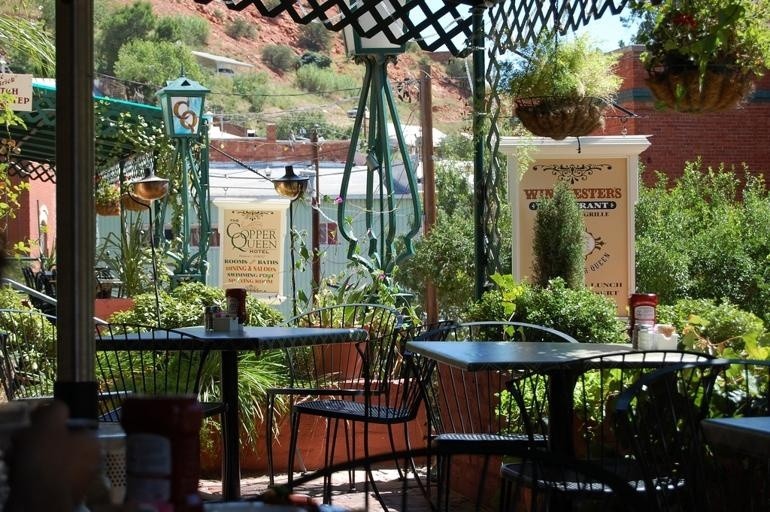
[402,321,581,511]
[607,359,770,512]
[94,322,223,423]
[289,319,456,511]
[265,303,403,483]
[3,308,56,401]
[500,352,719,510]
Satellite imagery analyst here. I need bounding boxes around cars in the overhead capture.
[347,106,369,119]
[215,68,235,76]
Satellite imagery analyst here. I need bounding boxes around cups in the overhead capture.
[225,288,247,319]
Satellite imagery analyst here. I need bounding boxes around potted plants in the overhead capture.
[511,0,770,142]
[92,179,149,215]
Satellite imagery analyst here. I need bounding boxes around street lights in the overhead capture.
[337,0,421,322]
[155,62,212,291]
